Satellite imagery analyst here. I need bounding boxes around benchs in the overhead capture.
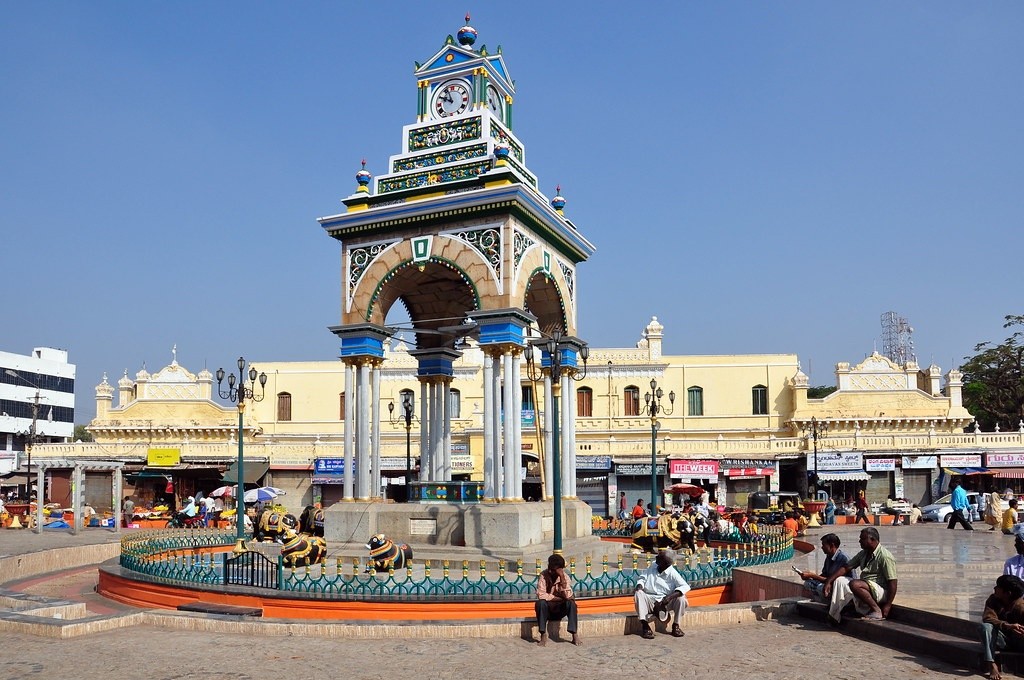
[870,502,912,526]
[986,494,1024,522]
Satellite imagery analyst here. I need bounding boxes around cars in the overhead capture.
[920,491,1006,522]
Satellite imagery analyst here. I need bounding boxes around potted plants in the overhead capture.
[799,499,827,528]
[3,499,31,530]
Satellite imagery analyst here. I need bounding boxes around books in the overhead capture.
[792,565,803,575]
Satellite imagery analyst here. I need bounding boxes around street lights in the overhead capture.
[5,369,42,432]
[215,356,268,557]
[16,425,46,522]
[524,326,591,557]
[801,416,829,502]
[632,377,676,528]
[387,392,414,499]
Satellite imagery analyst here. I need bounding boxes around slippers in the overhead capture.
[861,614,885,622]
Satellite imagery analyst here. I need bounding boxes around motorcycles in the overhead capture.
[165,510,206,528]
[746,491,811,526]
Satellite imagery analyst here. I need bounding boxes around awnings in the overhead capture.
[945,468,1024,478]
[219,461,269,488]
[817,469,872,482]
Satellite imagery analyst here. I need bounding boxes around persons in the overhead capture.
[801,533,857,606]
[824,527,898,620]
[981,575,1024,680]
[535,555,583,647]
[634,549,691,639]
[0,490,283,529]
[1003,524,1024,580]
[529,479,1024,543]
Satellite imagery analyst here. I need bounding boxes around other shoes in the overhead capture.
[673,625,685,636]
[989,527,995,530]
[643,628,655,639]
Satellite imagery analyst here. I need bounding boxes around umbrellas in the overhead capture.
[209,486,238,520]
[663,484,706,507]
[244,487,286,523]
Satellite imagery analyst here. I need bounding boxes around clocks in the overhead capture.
[486,82,505,124]
[430,77,474,120]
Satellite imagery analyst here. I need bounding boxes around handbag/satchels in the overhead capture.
[968,513,972,523]
